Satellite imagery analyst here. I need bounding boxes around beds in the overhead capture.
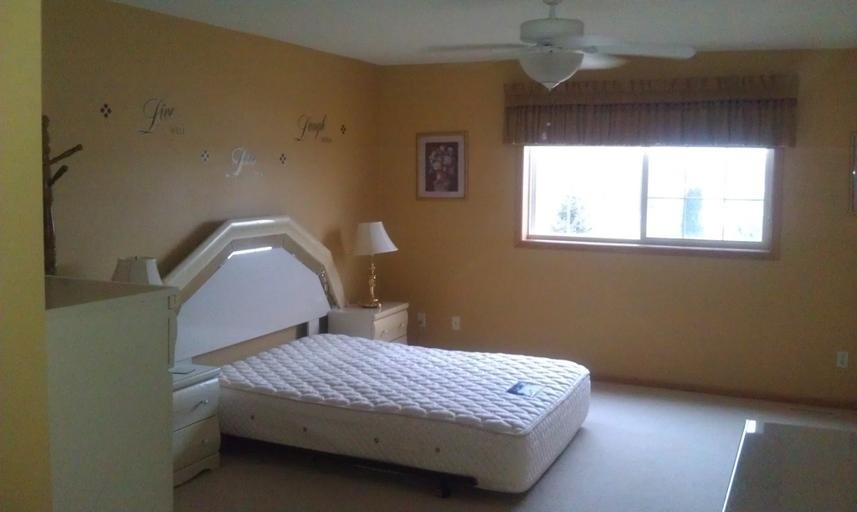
[163,214,589,497]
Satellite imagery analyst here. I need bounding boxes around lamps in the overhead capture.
[516,49,585,93]
[110,257,164,286]
[347,221,400,310]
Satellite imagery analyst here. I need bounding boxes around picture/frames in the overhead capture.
[413,131,469,200]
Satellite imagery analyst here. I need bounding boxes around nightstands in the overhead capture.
[327,302,409,345]
[170,363,222,488]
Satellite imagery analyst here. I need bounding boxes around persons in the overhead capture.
[426,143,458,192]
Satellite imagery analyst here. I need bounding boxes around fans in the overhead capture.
[410,1,695,70]
